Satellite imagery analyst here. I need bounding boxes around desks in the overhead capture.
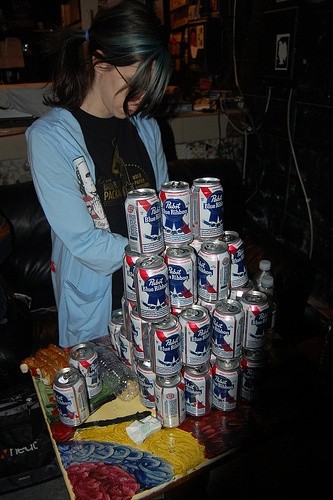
[29,333,252,499]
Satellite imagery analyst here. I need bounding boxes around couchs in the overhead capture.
[0,160,313,426]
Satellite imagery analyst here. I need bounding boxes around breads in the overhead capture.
[21,344,72,385]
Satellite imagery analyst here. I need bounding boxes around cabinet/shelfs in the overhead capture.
[167,0,333,230]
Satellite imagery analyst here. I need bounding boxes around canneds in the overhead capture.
[68,344,102,398]
[53,367,90,427]
[108,176,278,431]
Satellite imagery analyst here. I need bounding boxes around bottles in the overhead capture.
[251,260,274,290]
[257,276,276,307]
[263,301,276,352]
[85,341,140,402]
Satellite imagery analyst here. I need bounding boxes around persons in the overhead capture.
[23,0,175,349]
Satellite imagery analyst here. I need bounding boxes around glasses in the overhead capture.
[113,65,146,100]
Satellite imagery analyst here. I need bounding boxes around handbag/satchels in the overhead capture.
[0,387,63,496]
